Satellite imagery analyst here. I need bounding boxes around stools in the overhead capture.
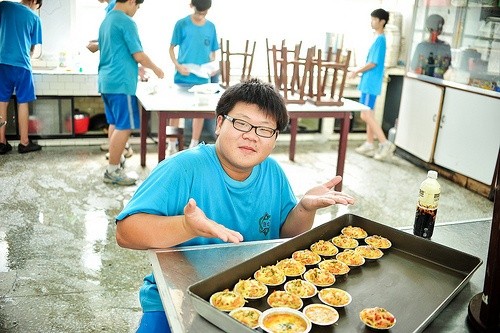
[217,35,353,107]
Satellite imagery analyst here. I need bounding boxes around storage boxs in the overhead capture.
[184,213,484,333]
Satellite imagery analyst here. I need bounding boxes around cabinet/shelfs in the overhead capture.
[392,75,500,200]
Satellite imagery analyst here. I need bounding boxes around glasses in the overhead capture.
[224,115,278,139]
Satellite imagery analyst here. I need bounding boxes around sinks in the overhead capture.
[31,65,58,70]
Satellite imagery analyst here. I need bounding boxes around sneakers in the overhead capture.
[104,147,133,160]
[101,144,111,151]
[375,140,396,160]
[0,139,12,155]
[18,140,41,153]
[355,142,376,157]
[104,168,137,185]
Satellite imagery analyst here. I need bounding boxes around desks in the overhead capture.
[134,81,374,192]
[144,218,500,333]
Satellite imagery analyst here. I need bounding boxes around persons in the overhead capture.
[410,13,451,80]
[348,8,396,160]
[97,0,164,185]
[0,0,43,155]
[166,0,220,157]
[114,78,357,333]
[87,0,134,159]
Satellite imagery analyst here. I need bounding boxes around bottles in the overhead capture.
[428,52,434,76]
[413,170,440,238]
